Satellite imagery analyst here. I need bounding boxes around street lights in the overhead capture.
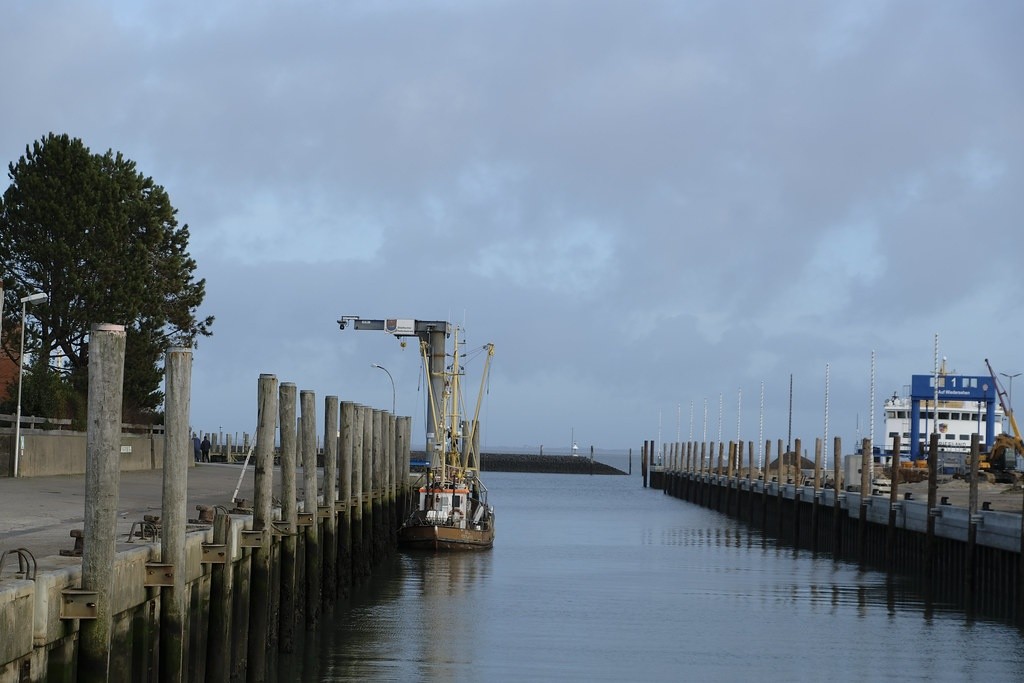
[13,293,48,476]
[368,363,396,416]
[998,372,1023,436]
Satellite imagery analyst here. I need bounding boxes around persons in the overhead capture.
[191,433,203,464]
[200,436,211,464]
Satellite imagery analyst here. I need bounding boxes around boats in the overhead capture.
[855,357,1005,475]
[398,326,495,550]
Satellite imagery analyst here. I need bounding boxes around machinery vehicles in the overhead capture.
[965,431,1023,484]
